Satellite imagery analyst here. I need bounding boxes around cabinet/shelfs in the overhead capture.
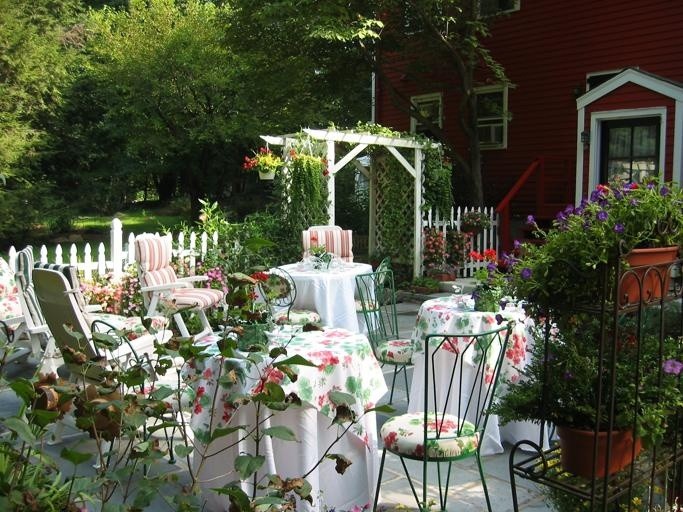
[506,237,682,510]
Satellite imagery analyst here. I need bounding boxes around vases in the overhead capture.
[482,171,682,317]
[258,169,275,180]
[433,273,456,280]
[604,245,681,305]
[237,322,268,352]
[473,287,499,313]
[554,422,643,475]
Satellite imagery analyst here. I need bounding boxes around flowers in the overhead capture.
[448,230,473,266]
[536,445,667,510]
[470,248,500,312]
[424,226,443,268]
[243,147,285,172]
[462,210,492,234]
[478,311,683,457]
[287,148,331,226]
[246,272,269,324]
[426,260,456,278]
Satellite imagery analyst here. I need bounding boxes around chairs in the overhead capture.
[0,254,27,351]
[15,244,127,351]
[355,269,414,406]
[34,270,172,384]
[303,225,354,262]
[355,256,391,345]
[370,322,514,512]
[256,266,320,326]
[133,233,225,340]
[91,321,193,477]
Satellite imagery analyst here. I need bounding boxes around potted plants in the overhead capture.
[308,243,331,269]
[407,275,441,293]
